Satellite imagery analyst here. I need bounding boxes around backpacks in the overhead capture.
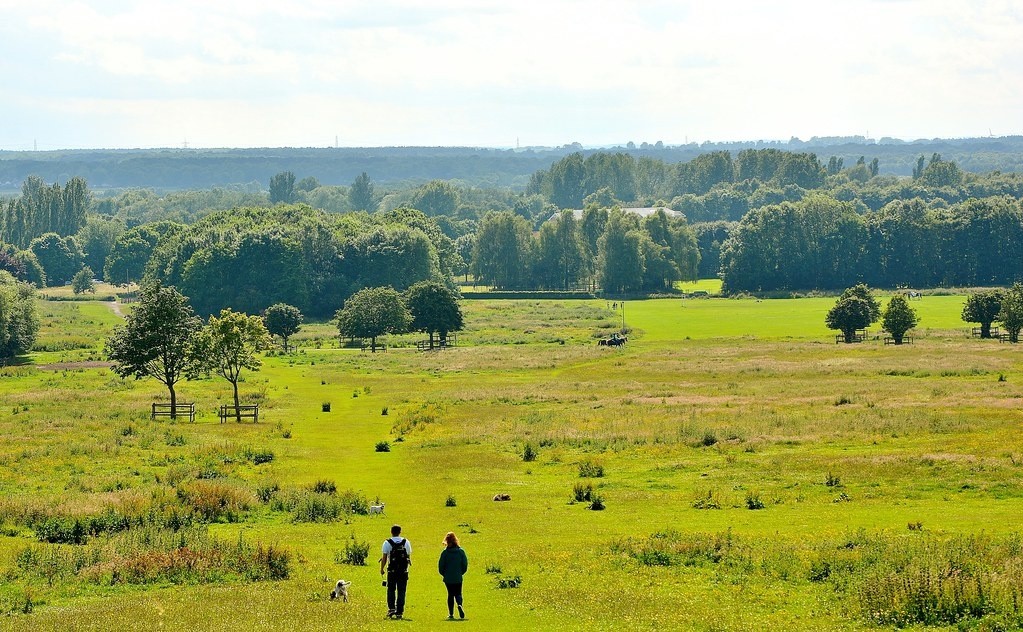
[385,536,410,577]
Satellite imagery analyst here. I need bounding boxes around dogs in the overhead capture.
[370,502,387,519]
[330,580,350,603]
[493,493,511,501]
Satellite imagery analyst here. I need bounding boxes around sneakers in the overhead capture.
[457,606,466,618]
[447,614,455,621]
[397,613,404,619]
[385,609,395,619]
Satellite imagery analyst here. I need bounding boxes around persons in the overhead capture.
[606,300,623,309]
[438,532,468,619]
[381,526,412,620]
